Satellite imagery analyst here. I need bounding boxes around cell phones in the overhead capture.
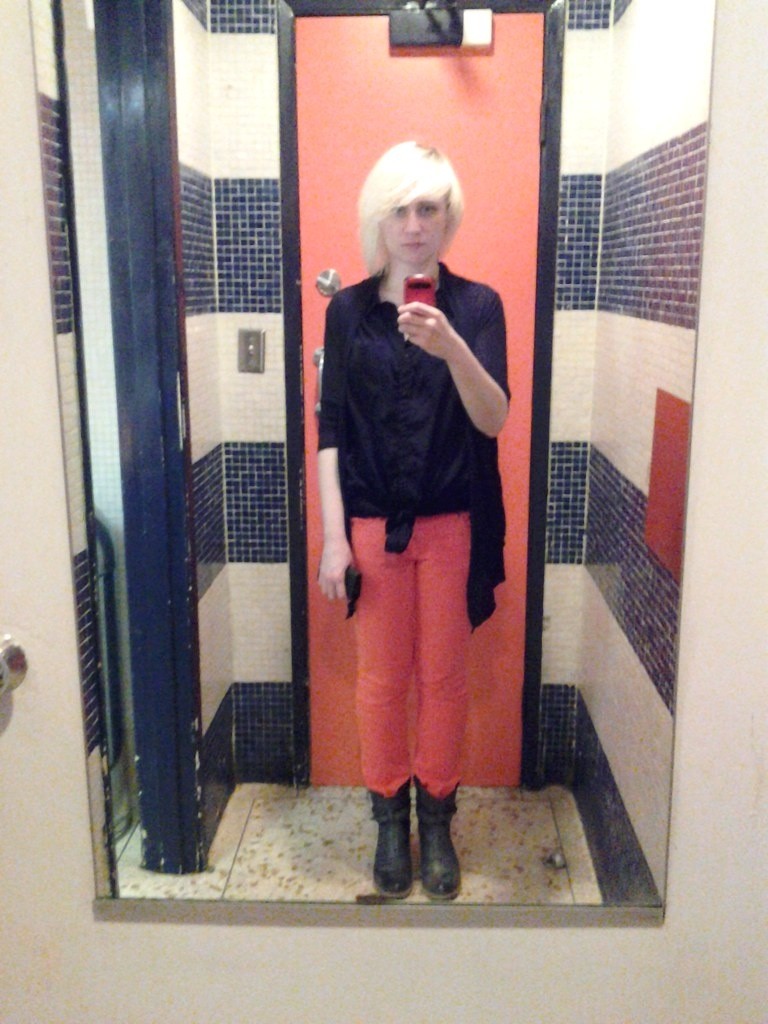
[403,274,436,309]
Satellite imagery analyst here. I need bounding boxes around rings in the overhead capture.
[404,334,409,342]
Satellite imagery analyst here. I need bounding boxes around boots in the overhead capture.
[371,779,414,899]
[413,774,462,900]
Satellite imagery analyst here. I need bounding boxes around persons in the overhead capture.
[315,139,512,900]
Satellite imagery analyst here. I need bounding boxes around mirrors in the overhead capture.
[48,0,716,930]
